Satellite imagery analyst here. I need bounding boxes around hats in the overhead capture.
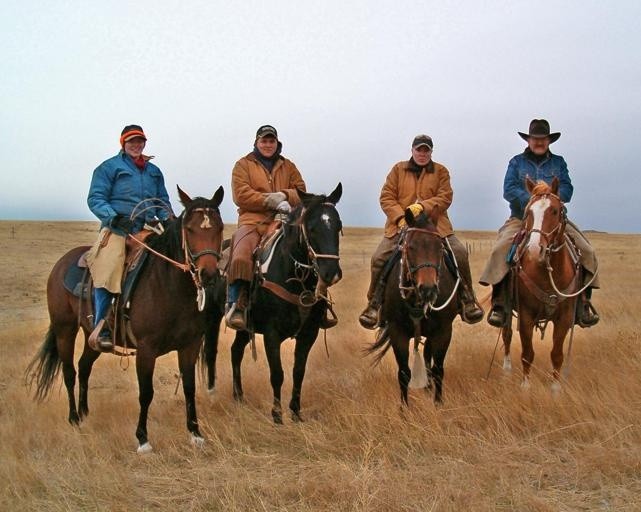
[256,125,277,137]
[517,119,561,144]
[120,125,144,145]
[412,134,432,149]
[122,133,146,141]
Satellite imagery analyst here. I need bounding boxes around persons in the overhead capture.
[223,122,307,330]
[357,133,485,328]
[85,123,175,350]
[482,117,602,327]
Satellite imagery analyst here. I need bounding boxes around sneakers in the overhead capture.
[97,336,112,347]
[489,311,505,325]
[359,308,377,326]
[461,302,482,321]
[575,310,599,323]
[229,311,244,328]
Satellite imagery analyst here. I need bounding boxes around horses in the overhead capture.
[498,173,581,398]
[22,185,225,457]
[361,203,460,413]
[195,181,343,429]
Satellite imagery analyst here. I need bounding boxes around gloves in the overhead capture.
[276,200,291,213]
[261,191,286,210]
[397,217,406,228]
[111,214,136,234]
[406,203,423,218]
